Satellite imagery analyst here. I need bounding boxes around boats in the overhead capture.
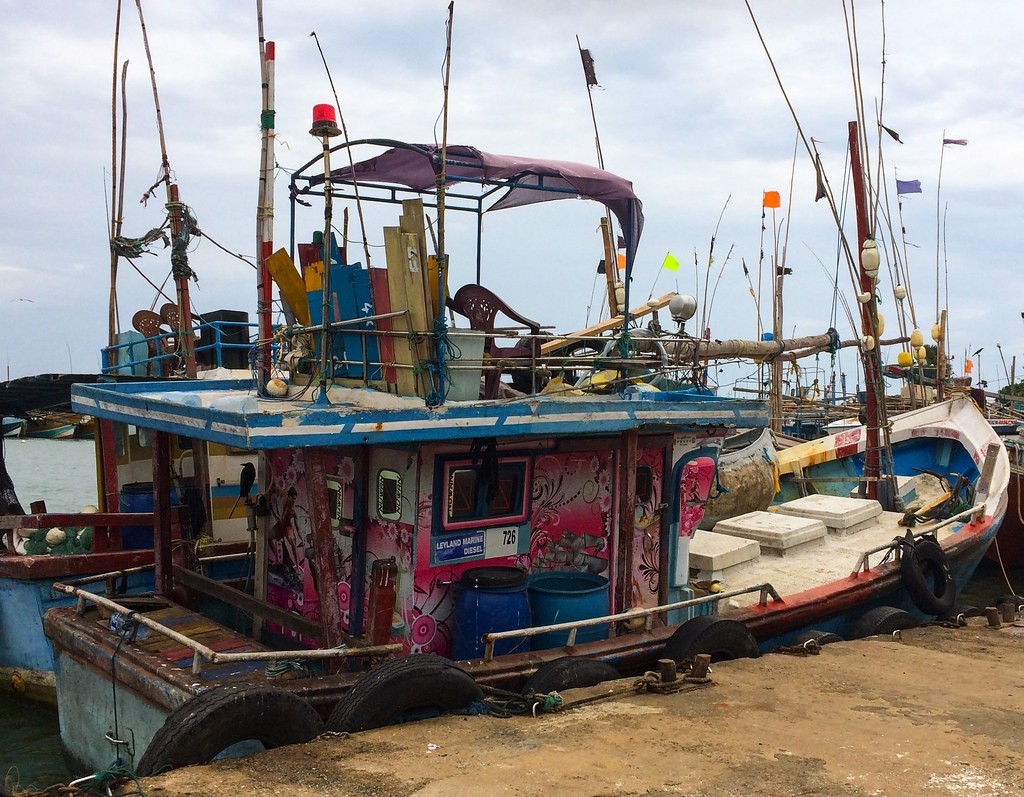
[0,2,1024,789]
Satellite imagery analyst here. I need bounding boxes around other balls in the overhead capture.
[267,378,288,397]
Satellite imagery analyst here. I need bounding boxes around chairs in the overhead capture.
[454,283,540,400]
[159,303,218,371]
[132,309,173,375]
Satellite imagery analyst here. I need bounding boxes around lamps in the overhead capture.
[670,294,698,380]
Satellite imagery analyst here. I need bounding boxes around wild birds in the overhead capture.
[239,462,255,497]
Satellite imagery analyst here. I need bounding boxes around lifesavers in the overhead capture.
[901,539,957,614]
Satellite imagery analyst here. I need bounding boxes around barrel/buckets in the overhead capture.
[120,482,180,550]
[452,566,530,660]
[526,571,610,651]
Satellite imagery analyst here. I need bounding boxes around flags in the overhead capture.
[762,191,782,208]
[618,254,626,269]
[597,260,605,274]
[965,360,973,373]
[897,180,922,193]
[618,236,626,248]
[663,254,680,273]
